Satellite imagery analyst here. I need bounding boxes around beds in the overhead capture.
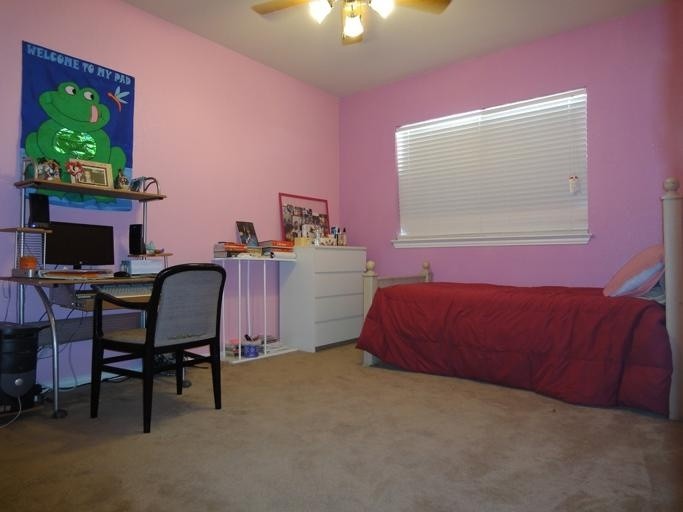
[363,177,683,419]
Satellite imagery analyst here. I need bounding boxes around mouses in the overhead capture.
[114,271,130,277]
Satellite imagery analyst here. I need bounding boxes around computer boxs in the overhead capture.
[0,321,39,414]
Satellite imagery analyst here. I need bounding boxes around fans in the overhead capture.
[251,0,453,46]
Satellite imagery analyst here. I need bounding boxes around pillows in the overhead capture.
[602,245,665,297]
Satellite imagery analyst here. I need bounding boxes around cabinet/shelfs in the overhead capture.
[0,179,173,258]
[212,257,300,365]
[279,244,368,353]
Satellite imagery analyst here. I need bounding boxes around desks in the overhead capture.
[1,276,190,418]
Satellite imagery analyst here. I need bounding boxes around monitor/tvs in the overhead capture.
[42,221,114,273]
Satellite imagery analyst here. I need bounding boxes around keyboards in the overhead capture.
[96,283,152,299]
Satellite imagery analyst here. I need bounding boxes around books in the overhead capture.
[214,239,297,258]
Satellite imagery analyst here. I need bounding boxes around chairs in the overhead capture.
[90,262,227,433]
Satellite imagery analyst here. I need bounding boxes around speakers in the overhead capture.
[129,224,146,254]
[28,193,50,223]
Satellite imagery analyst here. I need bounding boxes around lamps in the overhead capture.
[308,1,395,39]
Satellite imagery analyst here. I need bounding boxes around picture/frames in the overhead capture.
[278,192,331,242]
[69,159,114,190]
[236,221,259,246]
